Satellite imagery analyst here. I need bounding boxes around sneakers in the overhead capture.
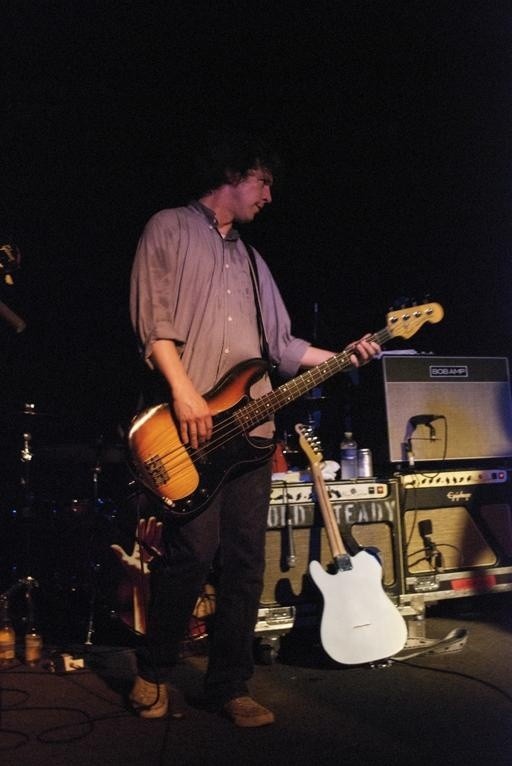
[224,693,273,729]
[131,678,167,720]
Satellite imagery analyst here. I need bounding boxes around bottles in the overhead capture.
[340,433,360,479]
[1,603,18,671]
[406,594,426,641]
[23,606,43,665]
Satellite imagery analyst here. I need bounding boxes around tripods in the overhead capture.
[0,507,58,665]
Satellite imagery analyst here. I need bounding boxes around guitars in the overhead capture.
[296,423,408,665]
[118,301,445,517]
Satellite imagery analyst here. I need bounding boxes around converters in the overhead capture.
[57,653,84,672]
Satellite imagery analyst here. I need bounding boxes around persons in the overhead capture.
[108,516,164,637]
[127,142,384,727]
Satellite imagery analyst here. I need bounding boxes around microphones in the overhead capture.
[409,413,443,429]
[285,506,297,568]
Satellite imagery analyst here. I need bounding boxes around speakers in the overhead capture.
[369,355,512,471]
[254,477,407,666]
[397,464,512,621]
